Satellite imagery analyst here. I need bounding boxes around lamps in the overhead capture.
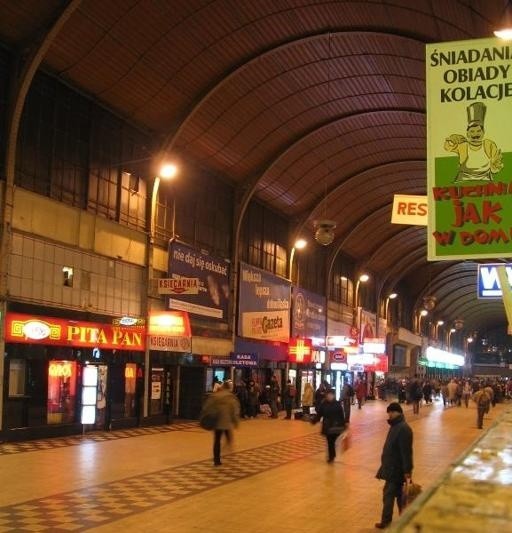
[422,261,465,331]
[311,0,338,246]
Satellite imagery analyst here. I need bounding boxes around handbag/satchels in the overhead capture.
[401,475,423,510]
[200,411,218,431]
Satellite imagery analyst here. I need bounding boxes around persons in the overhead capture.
[200,379,241,465]
[374,401,413,530]
[440,123,502,183]
[311,388,345,462]
[234,370,511,430]
